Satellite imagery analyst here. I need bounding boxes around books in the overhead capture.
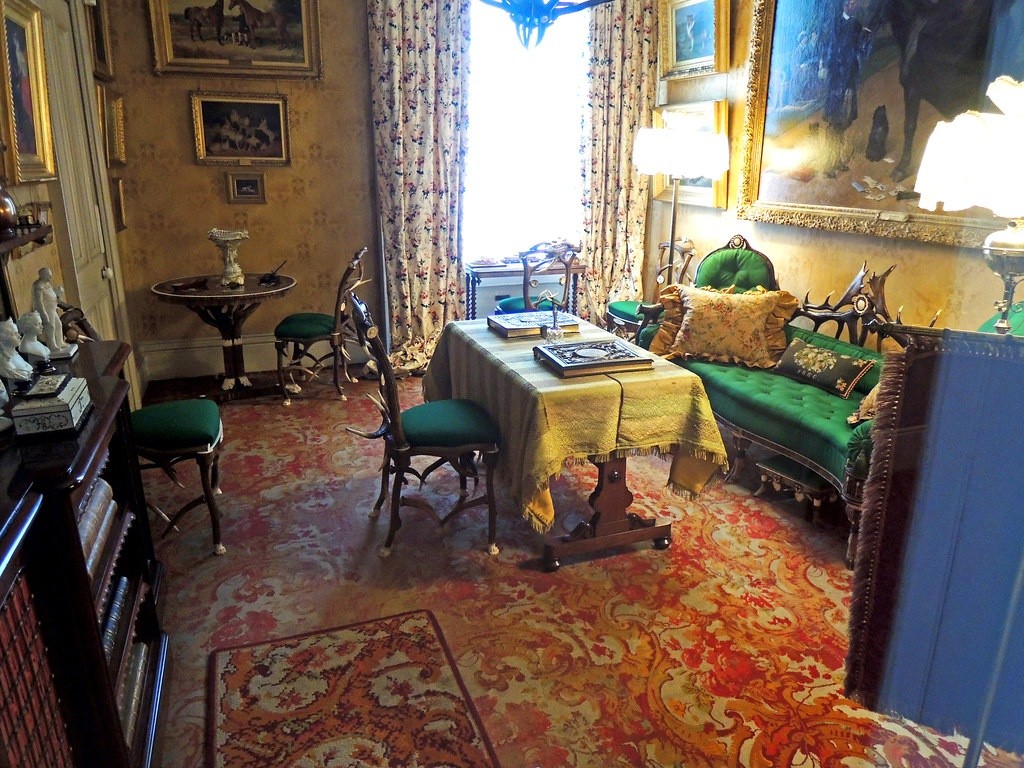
[533,339,655,379]
[487,310,579,340]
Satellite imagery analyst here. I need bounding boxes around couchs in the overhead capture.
[628,235,1024,571]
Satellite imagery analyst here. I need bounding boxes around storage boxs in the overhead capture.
[11,377,91,436]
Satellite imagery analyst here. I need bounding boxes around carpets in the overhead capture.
[204,608,503,768]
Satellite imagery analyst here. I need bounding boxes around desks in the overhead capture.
[151,272,298,405]
[419,309,730,572]
[464,261,587,321]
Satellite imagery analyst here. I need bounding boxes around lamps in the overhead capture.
[913,74,1024,335]
[631,108,731,287]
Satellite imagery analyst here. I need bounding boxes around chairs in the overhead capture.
[58,299,228,557]
[343,291,503,559]
[605,236,697,340]
[495,235,584,315]
[273,244,373,406]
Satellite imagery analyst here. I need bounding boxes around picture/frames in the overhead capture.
[108,175,128,234]
[145,0,327,83]
[84,0,117,83]
[188,89,293,168]
[657,0,731,83]
[95,82,129,170]
[652,96,730,210]
[0,0,58,189]
[735,0,1024,250]
[224,171,268,204]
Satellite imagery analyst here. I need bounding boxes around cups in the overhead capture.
[544,327,566,346]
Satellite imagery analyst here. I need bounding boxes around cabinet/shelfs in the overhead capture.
[0,338,175,768]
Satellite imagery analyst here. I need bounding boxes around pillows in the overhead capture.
[649,282,799,369]
[770,336,877,399]
[845,382,881,425]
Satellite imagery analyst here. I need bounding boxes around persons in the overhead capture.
[0,317,33,380]
[17,310,50,357]
[33,268,70,350]
